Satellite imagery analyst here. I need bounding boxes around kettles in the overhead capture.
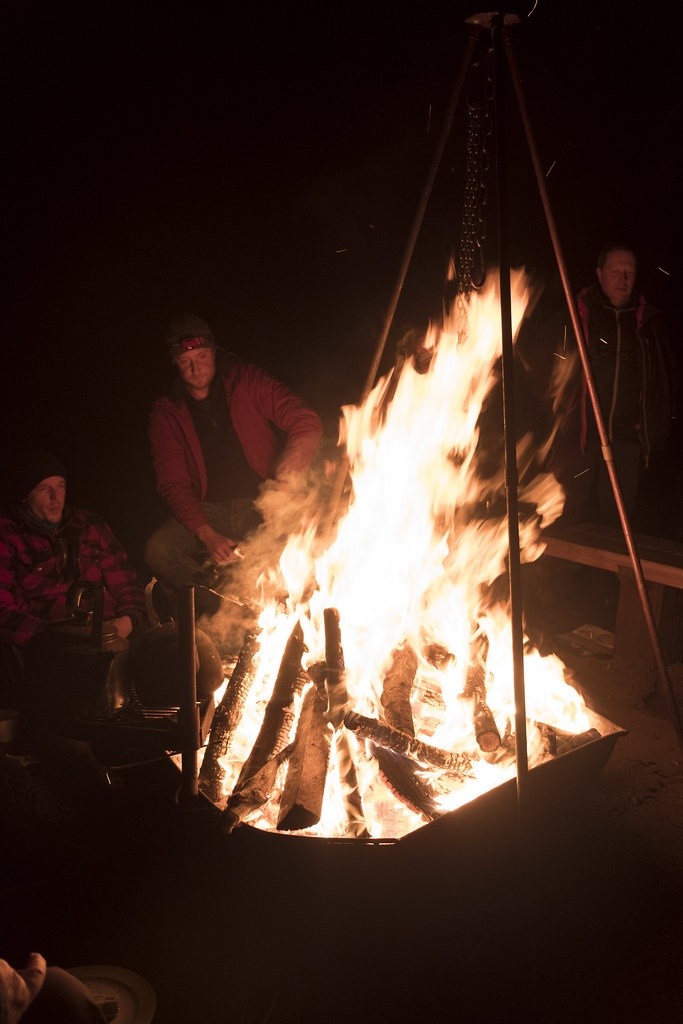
[130,573,225,706]
[28,578,134,721]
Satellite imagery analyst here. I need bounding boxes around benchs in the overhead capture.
[535,521,682,705]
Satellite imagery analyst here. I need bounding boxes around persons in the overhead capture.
[0,463,144,708]
[537,247,676,613]
[141,316,325,628]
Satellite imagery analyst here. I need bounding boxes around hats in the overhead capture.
[14,448,67,503]
[164,313,217,357]
